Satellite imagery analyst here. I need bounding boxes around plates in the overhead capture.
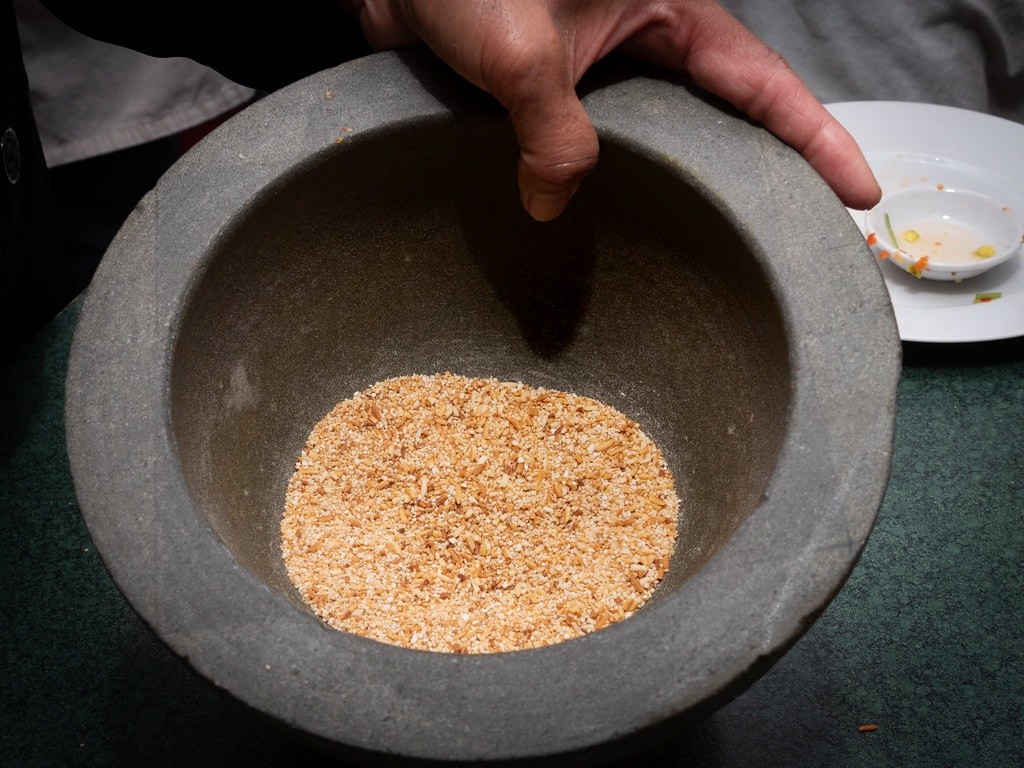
[865,184,1024,282]
[775,100,1023,345]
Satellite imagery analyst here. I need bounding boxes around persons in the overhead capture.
[1,0,881,346]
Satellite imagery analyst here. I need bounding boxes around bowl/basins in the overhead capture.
[64,36,904,768]
